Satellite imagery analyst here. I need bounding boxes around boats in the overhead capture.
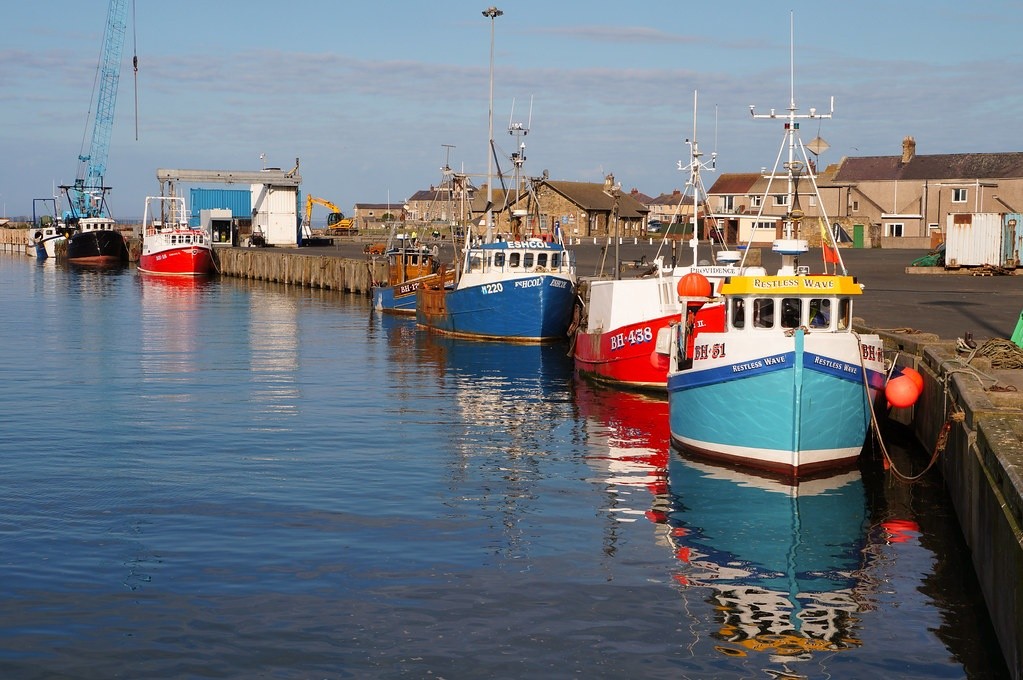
[570,367,700,652]
[666,11,887,480]
[411,323,569,560]
[56,178,130,265]
[26,198,67,258]
[573,87,749,388]
[365,225,459,321]
[413,96,579,346]
[662,443,877,680]
[137,196,212,278]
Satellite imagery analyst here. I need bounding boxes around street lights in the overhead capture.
[441,144,456,168]
[482,7,504,244]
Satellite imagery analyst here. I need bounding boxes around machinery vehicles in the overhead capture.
[306,194,355,237]
[62,0,138,222]
